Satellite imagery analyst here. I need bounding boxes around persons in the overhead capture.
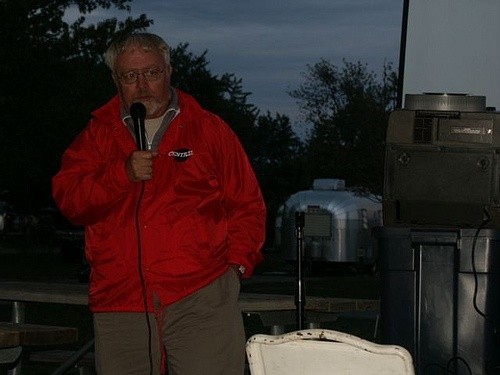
[50,30,266,374]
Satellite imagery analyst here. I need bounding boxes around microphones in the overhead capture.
[130,102,146,151]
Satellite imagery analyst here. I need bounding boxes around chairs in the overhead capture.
[246,328,415,375]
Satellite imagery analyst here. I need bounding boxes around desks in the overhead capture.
[0,279,382,375]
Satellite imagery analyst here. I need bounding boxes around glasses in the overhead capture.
[115,66,166,85]
